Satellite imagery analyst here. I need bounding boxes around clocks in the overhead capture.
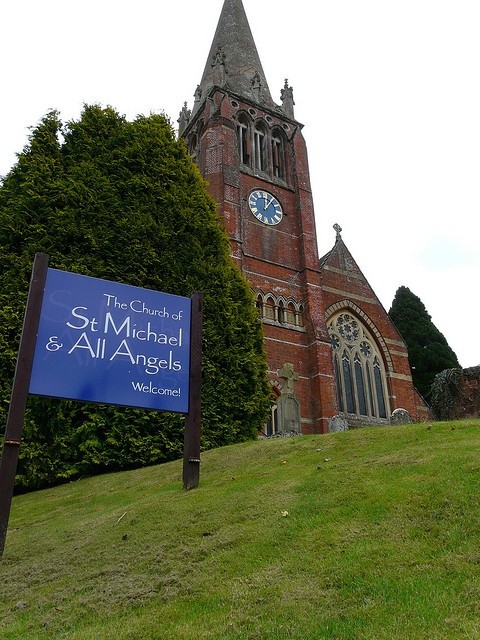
[249,190,283,226]
